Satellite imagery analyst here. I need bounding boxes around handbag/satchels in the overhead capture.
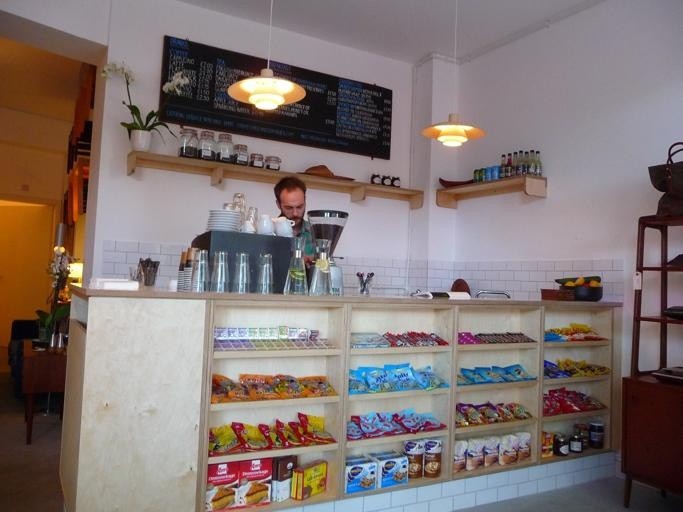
[648,142,683,217]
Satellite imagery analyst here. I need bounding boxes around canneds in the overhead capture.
[589,421,604,449]
[474,169,480,182]
[569,433,583,454]
[492,165,499,180]
[370,173,400,188]
[486,166,491,181]
[554,438,569,456]
[480,167,486,181]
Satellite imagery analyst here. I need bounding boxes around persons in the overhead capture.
[273,176,314,264]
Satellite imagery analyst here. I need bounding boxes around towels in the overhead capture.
[418,291,472,302]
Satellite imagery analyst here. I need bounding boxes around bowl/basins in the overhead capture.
[559,286,603,301]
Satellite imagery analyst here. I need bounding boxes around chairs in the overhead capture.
[21,338,66,447]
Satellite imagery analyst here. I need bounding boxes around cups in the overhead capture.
[176,247,274,295]
[222,191,257,223]
[141,260,160,286]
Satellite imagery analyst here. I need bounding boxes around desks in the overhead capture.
[8,319,64,416]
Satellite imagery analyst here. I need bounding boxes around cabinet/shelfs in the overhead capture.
[453,300,543,479]
[199,298,347,512]
[619,213,683,512]
[541,302,622,460]
[342,298,451,500]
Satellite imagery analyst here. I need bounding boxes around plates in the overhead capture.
[540,289,576,301]
[206,209,242,234]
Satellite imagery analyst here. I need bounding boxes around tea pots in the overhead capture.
[255,214,295,238]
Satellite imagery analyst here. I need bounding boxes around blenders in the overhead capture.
[306,209,349,296]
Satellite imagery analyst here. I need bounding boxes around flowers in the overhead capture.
[36,246,71,332]
[100,60,191,146]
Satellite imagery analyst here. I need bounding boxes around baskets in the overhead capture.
[541,289,576,301]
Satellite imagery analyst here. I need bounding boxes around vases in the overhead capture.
[49,334,64,353]
[128,129,152,152]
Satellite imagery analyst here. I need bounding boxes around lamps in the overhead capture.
[67,262,83,283]
[420,0,485,149]
[227,0,307,112]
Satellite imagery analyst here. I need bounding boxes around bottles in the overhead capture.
[177,128,281,172]
[575,423,590,448]
[283,236,308,296]
[499,149,542,178]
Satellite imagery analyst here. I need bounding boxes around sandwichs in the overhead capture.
[244,482,268,504]
[210,488,235,510]
